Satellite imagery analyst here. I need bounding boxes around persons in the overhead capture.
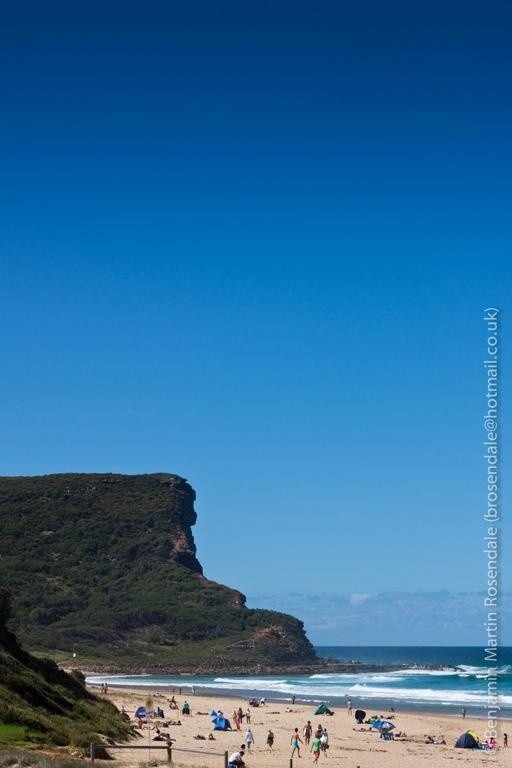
[503,731,509,747]
[461,704,466,719]
[424,734,447,745]
[484,739,493,748]
[490,736,497,749]
[100,680,408,767]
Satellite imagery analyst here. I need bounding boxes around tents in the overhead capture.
[454,726,480,749]
